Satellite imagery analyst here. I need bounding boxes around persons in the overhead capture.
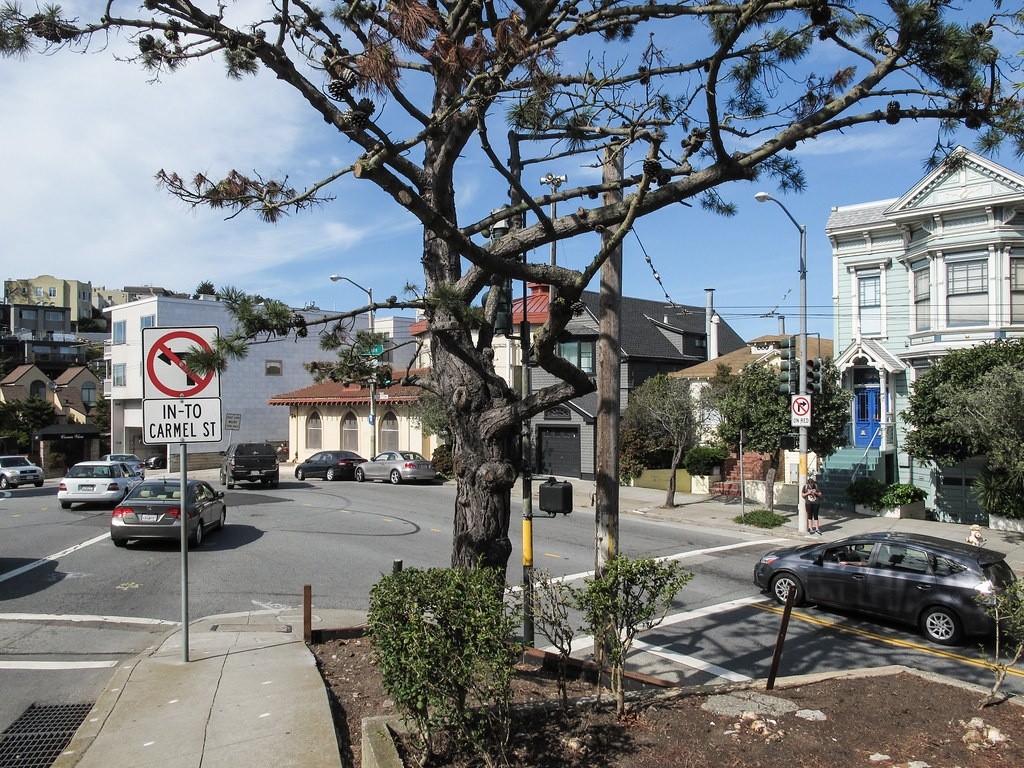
[802,479,822,535]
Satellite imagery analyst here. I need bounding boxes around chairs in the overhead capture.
[873,546,892,568]
[139,491,181,499]
[888,545,907,568]
[389,455,421,460]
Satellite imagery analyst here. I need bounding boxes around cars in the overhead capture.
[92,454,146,481]
[295,450,368,481]
[0,456,46,490]
[110,475,227,548]
[753,530,1020,646]
[142,454,167,470]
[354,450,436,485]
[57,460,144,510]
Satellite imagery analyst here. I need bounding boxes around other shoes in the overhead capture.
[816,529,822,535]
[808,527,815,534]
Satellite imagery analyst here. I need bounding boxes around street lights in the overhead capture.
[756,191,810,539]
[330,274,377,459]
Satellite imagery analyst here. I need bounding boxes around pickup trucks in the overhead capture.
[219,441,280,488]
